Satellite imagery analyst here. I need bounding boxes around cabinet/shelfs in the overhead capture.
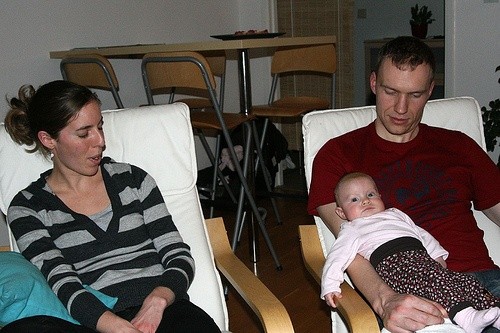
[363,39,445,105]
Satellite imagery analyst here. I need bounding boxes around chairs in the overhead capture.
[160,51,226,220]
[296,95,500,333]
[59,54,123,109]
[0,102,294,333]
[138,53,284,301]
[251,43,338,199]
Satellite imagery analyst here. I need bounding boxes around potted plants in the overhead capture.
[409,4,435,39]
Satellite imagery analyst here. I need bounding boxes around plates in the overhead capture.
[210,32,287,40]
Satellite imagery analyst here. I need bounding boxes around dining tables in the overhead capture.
[47,35,337,263]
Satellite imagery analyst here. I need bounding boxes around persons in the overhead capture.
[319,172,500,333]
[0,80,222,333]
[305,35,500,333]
